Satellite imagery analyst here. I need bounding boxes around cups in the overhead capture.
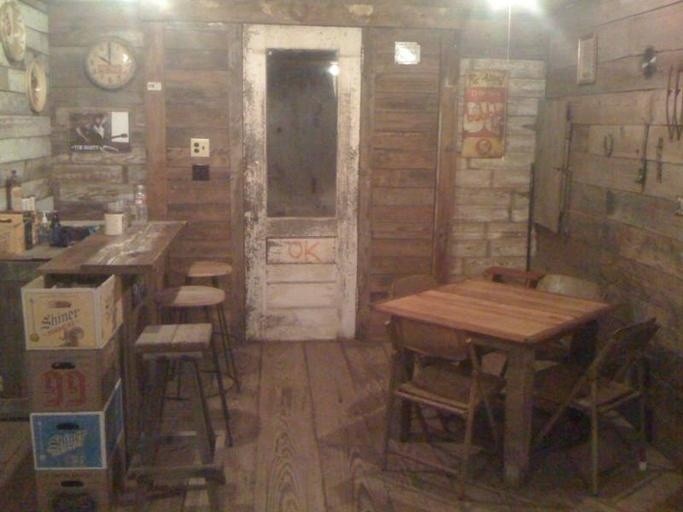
[102,212,122,237]
[106,199,131,230]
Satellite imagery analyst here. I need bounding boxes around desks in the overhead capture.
[376,280,618,487]
[33,215,188,280]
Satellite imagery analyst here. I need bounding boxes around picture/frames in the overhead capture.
[576,32,597,85]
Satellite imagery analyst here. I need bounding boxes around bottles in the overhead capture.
[6,168,24,214]
[132,182,148,228]
[19,206,71,248]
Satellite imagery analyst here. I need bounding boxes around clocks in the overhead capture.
[83,36,138,91]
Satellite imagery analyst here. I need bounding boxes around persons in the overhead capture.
[92,114,109,139]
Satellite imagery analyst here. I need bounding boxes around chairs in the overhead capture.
[379,315,504,501]
[389,274,489,369]
[533,317,661,496]
[529,274,607,376]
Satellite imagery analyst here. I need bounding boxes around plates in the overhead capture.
[0,0,27,62]
[26,55,47,113]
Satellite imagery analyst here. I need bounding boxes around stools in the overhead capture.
[164,259,240,400]
[121,323,224,512]
[146,285,236,455]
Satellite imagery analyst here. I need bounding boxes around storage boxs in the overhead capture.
[22,277,124,511]
